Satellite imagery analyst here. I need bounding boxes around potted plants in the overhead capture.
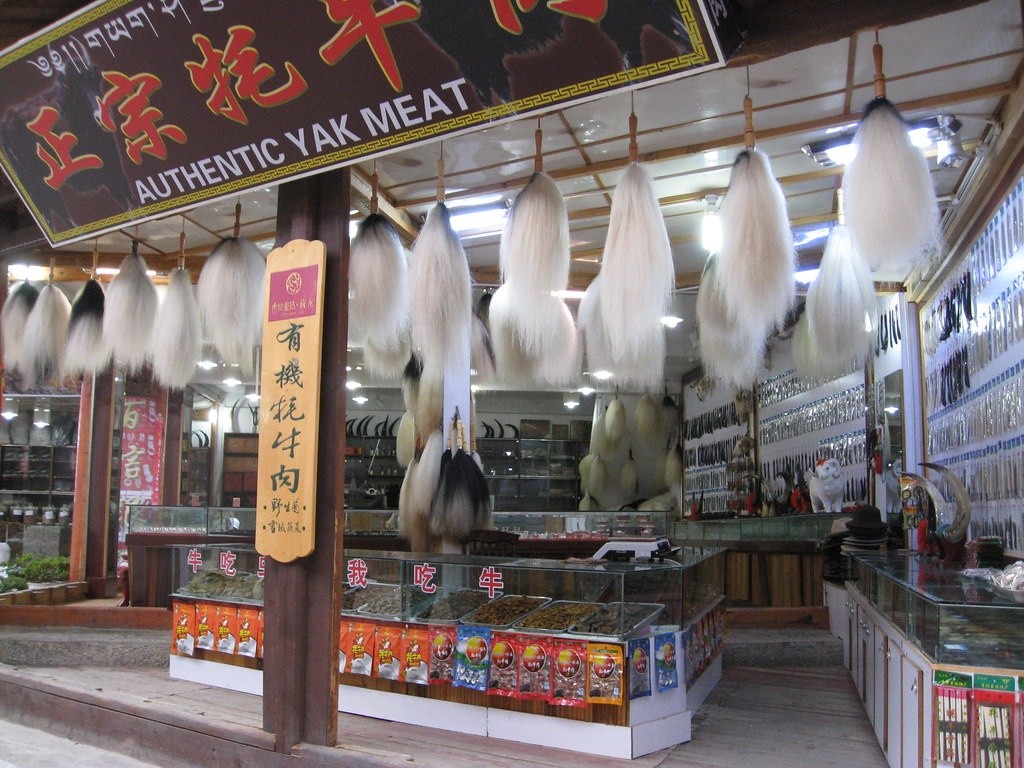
[0,552,87,607]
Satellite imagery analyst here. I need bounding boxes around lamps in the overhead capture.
[418,199,513,231]
[221,366,246,386]
[927,114,967,172]
[662,293,684,329]
[245,385,261,402]
[0,397,19,420]
[196,344,219,370]
[33,398,51,428]
[352,388,368,404]
[800,110,963,168]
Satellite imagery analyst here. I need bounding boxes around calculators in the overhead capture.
[671,546,681,556]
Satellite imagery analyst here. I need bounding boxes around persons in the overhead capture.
[776,463,805,508]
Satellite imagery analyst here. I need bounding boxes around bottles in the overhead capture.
[42,506,56,524]
[0,506,38,524]
[58,504,70,523]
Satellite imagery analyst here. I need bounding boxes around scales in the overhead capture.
[592,534,672,563]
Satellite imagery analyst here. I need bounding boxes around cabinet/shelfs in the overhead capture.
[108,428,190,505]
[520,438,591,512]
[186,447,210,507]
[0,443,77,507]
[221,433,258,508]
[842,550,1024,768]
[344,435,521,513]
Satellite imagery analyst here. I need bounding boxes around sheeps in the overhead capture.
[810,459,846,513]
